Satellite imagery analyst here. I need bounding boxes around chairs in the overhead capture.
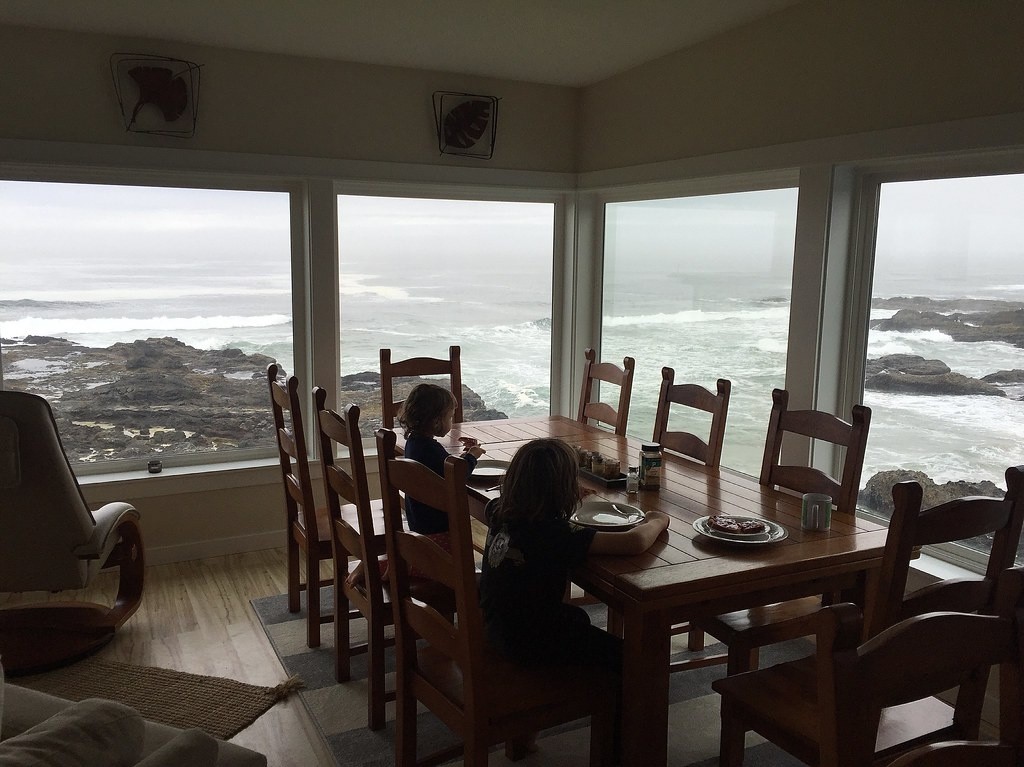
[0,389,146,675]
[264,346,610,767]
[576,345,1024,767]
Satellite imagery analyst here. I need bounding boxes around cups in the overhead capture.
[801,493,832,532]
[147,460,162,472]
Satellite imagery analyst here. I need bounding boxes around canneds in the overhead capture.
[639,442,662,486]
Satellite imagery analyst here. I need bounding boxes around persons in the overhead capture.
[343,384,486,625]
[479,438,670,674]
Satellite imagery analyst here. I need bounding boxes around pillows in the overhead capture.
[0,697,146,767]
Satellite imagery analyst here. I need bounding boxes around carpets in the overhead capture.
[250,558,817,766]
[5,654,308,743]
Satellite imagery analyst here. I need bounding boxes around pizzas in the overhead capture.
[458,437,478,448]
[708,515,741,535]
[582,486,597,496]
[738,520,765,533]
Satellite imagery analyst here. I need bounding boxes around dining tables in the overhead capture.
[383,414,922,767]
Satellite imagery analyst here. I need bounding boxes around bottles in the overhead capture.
[639,442,662,491]
[626,466,639,494]
[575,445,621,480]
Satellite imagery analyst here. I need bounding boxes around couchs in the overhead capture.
[0,683,270,767]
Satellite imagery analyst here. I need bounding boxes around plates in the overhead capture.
[470,460,511,482]
[692,514,789,544]
[567,501,645,531]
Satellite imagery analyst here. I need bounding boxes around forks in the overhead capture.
[612,504,646,520]
